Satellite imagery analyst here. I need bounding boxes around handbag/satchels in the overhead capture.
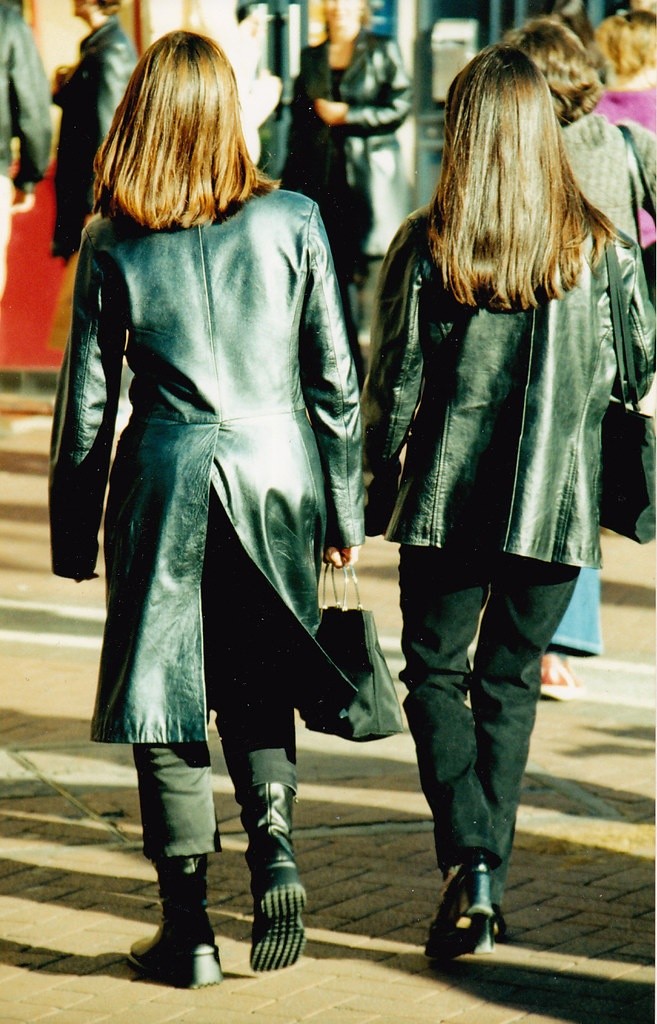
[601,397,655,548]
[354,134,407,259]
[289,552,403,742]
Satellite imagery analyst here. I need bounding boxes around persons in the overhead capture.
[502,17,657,704]
[49,0,143,270]
[590,9,657,309]
[281,2,413,395]
[49,32,365,992]
[0,1,51,299]
[362,45,657,961]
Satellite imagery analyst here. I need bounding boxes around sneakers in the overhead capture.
[537,655,582,702]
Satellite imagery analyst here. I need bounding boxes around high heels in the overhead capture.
[423,854,494,961]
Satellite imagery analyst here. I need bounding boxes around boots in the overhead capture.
[128,856,224,988]
[235,781,308,975]
